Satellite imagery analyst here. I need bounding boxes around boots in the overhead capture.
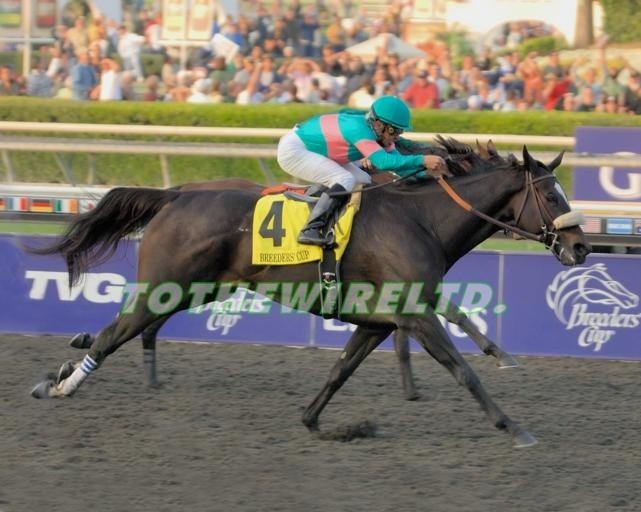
[297,192,340,246]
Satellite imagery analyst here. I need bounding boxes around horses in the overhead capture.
[22,135,590,448]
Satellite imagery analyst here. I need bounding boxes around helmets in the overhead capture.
[371,95,411,131]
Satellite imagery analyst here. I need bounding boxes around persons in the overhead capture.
[276,95,449,246]
